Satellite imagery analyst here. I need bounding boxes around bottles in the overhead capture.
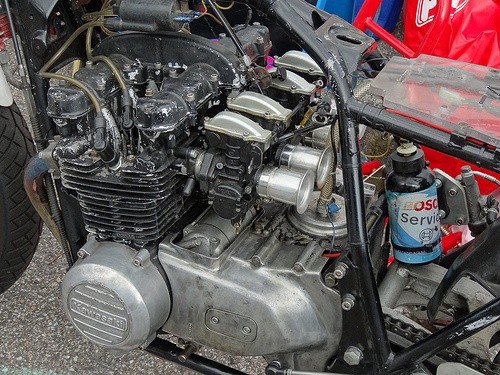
[385,149,442,265]
